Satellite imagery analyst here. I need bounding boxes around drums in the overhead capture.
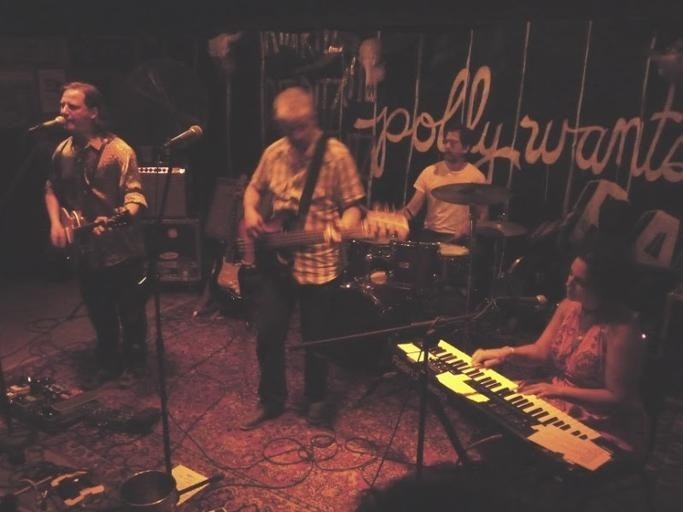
[357,239,402,262]
[434,244,471,284]
[385,239,441,292]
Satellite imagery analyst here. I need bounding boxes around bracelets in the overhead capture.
[509,346,514,363]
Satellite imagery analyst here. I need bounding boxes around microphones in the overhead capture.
[28,116,66,134]
[496,294,547,309]
[164,125,203,150]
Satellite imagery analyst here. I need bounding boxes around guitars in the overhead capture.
[233,201,405,271]
[54,208,135,255]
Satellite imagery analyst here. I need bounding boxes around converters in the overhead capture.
[126,407,159,435]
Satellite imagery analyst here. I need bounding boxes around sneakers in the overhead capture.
[238,406,268,431]
[305,400,327,423]
[251,429,253,430]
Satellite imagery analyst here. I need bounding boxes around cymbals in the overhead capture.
[476,219,529,239]
[431,183,515,206]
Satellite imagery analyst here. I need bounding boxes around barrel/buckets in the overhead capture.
[120,470,179,512]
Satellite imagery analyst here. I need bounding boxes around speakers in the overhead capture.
[207,176,248,243]
[139,162,209,219]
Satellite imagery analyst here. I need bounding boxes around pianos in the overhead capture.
[392,334,627,490]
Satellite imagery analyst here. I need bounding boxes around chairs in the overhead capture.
[606,354,666,512]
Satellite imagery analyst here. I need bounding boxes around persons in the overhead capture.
[41,79,151,392]
[467,247,655,484]
[235,85,367,434]
[390,122,493,243]
